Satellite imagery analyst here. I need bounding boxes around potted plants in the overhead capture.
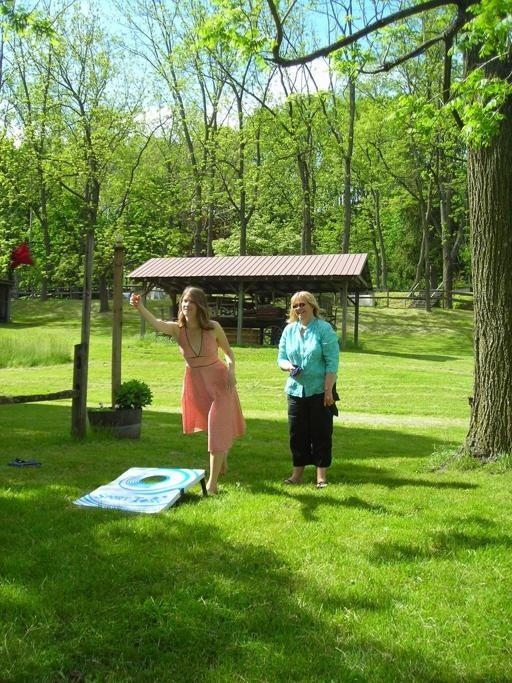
[87,377,154,439]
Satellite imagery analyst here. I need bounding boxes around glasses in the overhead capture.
[291,303,306,309]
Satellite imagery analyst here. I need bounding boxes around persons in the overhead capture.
[129,286,246,494]
[276,291,340,489]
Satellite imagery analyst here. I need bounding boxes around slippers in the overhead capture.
[316,480,328,489]
